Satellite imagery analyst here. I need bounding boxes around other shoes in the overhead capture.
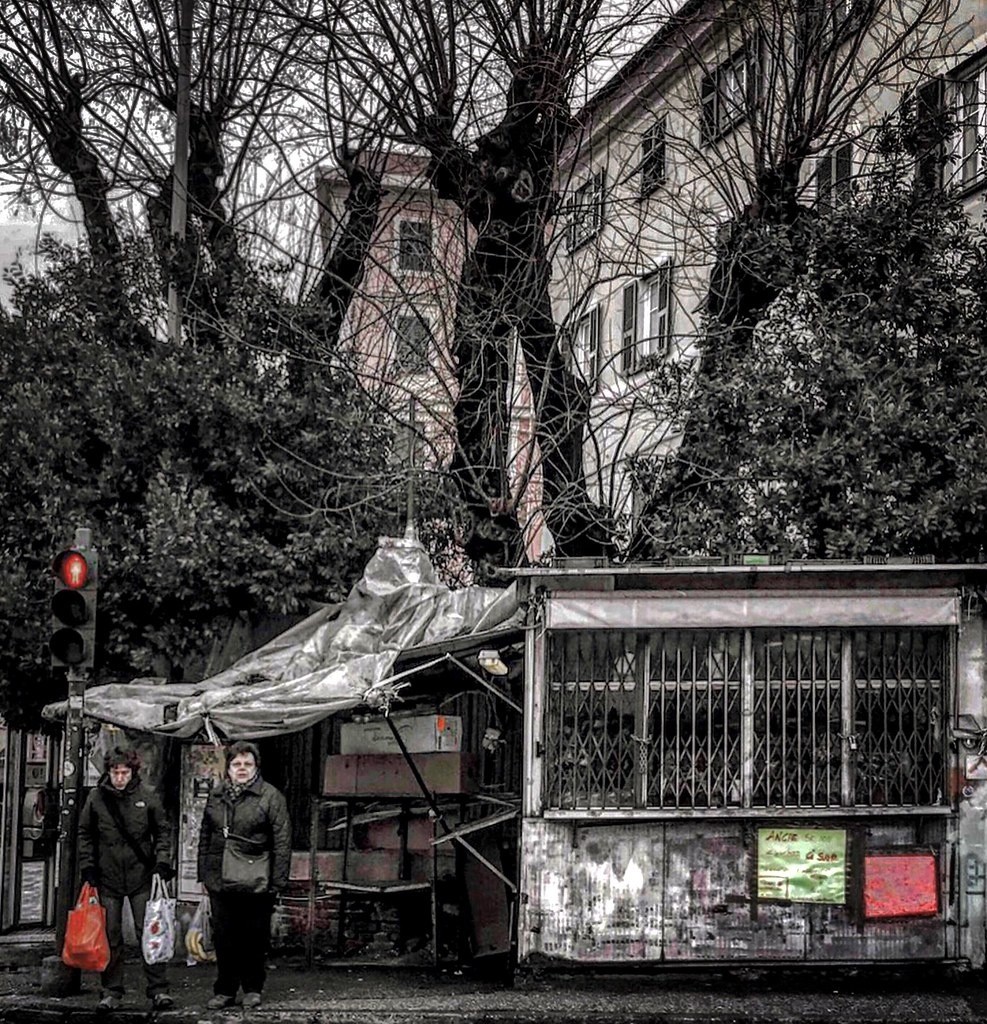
[96,995,121,1010]
[153,993,174,1008]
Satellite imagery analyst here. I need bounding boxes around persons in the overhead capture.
[77,746,172,1012]
[196,742,290,1010]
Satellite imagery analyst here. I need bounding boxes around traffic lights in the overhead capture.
[48,546,95,673]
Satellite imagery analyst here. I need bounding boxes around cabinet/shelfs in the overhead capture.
[300,792,472,969]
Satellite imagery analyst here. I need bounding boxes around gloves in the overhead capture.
[153,863,173,881]
[81,867,99,886]
[271,884,285,897]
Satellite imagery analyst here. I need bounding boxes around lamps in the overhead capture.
[473,642,525,676]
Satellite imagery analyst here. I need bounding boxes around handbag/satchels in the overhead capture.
[61,882,111,972]
[220,803,272,894]
[141,873,177,965]
[184,882,217,963]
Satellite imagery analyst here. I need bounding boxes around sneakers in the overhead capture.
[207,994,235,1009]
[242,992,261,1007]
